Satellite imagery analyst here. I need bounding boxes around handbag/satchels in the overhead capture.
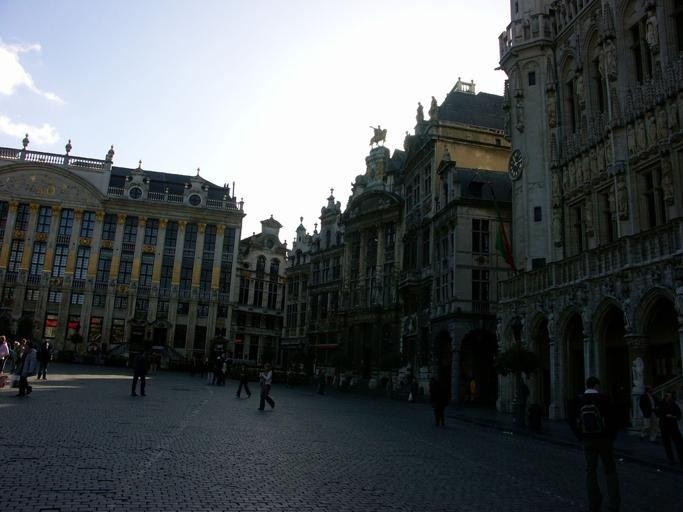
[11,375,20,388]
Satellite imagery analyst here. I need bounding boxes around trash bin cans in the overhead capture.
[526,403,543,431]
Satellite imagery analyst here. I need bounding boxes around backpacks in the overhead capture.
[575,394,604,438]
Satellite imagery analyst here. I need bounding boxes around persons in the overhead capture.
[149,352,157,376]
[409,377,418,404]
[511,378,542,431]
[207,351,231,386]
[639,384,683,472]
[131,349,151,396]
[234,362,251,398]
[257,362,275,410]
[429,373,452,427]
[470,375,479,404]
[576,375,620,512]
[0,335,52,397]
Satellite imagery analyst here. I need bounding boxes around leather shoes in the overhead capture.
[16,390,32,397]
